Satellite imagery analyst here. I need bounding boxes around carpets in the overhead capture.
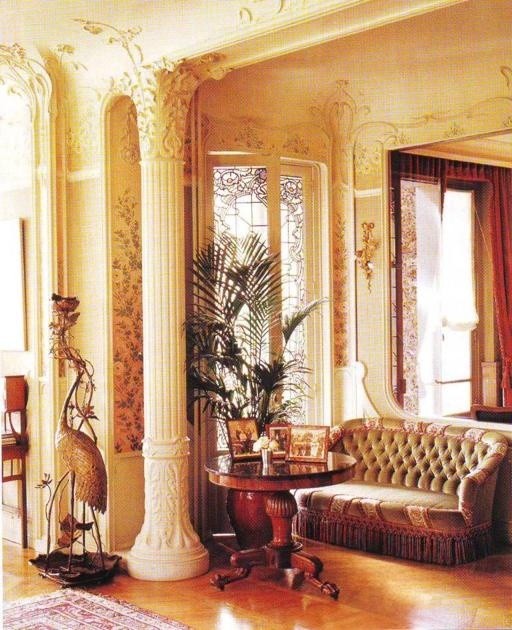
[4,587,195,630]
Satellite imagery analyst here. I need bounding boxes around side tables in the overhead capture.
[203,452,357,600]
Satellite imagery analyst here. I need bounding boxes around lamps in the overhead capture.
[2,350,30,435]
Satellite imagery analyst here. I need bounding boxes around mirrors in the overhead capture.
[386,127,512,423]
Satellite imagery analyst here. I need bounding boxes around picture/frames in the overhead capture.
[226,417,262,462]
[265,423,290,463]
[288,424,329,463]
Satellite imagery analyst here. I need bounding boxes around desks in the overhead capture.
[3,443,28,550]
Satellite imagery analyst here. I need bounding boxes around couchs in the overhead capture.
[290,416,509,566]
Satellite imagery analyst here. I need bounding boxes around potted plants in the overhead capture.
[183,231,329,551]
